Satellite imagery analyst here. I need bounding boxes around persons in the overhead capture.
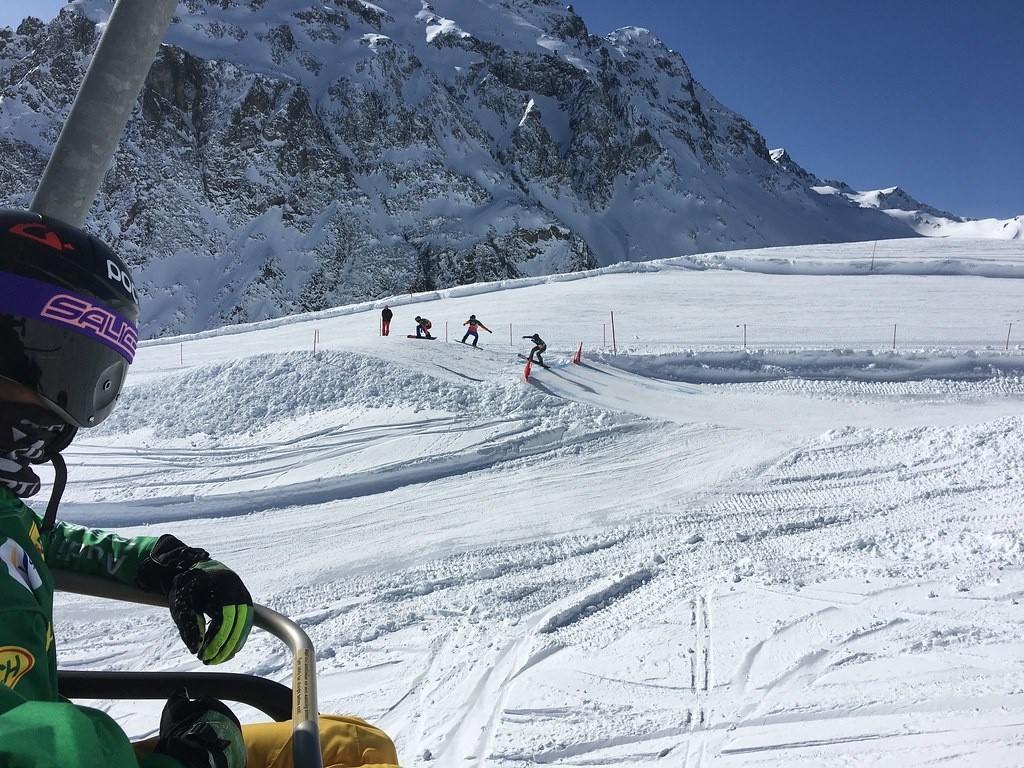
[462,315,492,346]
[381,304,393,336]
[415,316,432,338]
[522,333,546,364]
[0,205,402,766]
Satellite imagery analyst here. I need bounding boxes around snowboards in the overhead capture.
[407,334,437,340]
[518,353,550,369]
[453,338,483,351]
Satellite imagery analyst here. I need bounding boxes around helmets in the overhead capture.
[0,207,138,426]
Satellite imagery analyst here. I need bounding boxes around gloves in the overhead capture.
[138,533,254,665]
[151,685,247,766]
